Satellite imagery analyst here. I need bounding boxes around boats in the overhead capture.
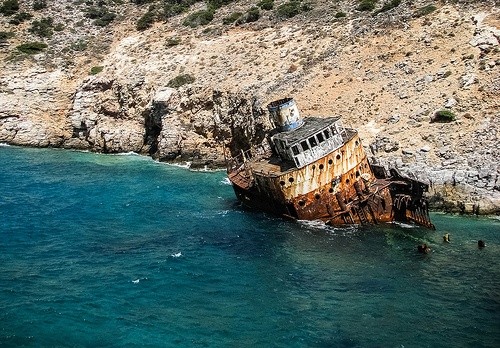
[222,96,437,232]
